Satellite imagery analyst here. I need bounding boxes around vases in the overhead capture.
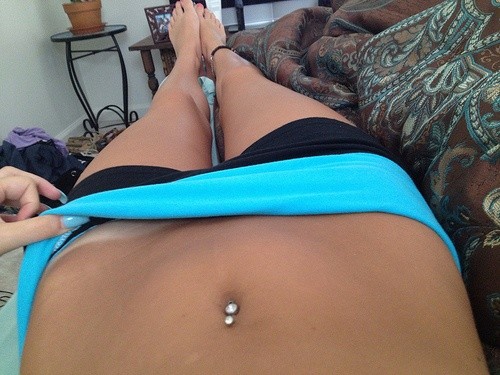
[63,0,101,29]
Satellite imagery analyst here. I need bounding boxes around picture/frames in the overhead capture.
[145,6,173,43]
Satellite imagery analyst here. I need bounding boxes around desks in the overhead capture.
[51,23,139,138]
[128,35,160,99]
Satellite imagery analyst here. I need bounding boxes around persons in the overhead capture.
[157,14,170,33]
[0,0,489,375]
